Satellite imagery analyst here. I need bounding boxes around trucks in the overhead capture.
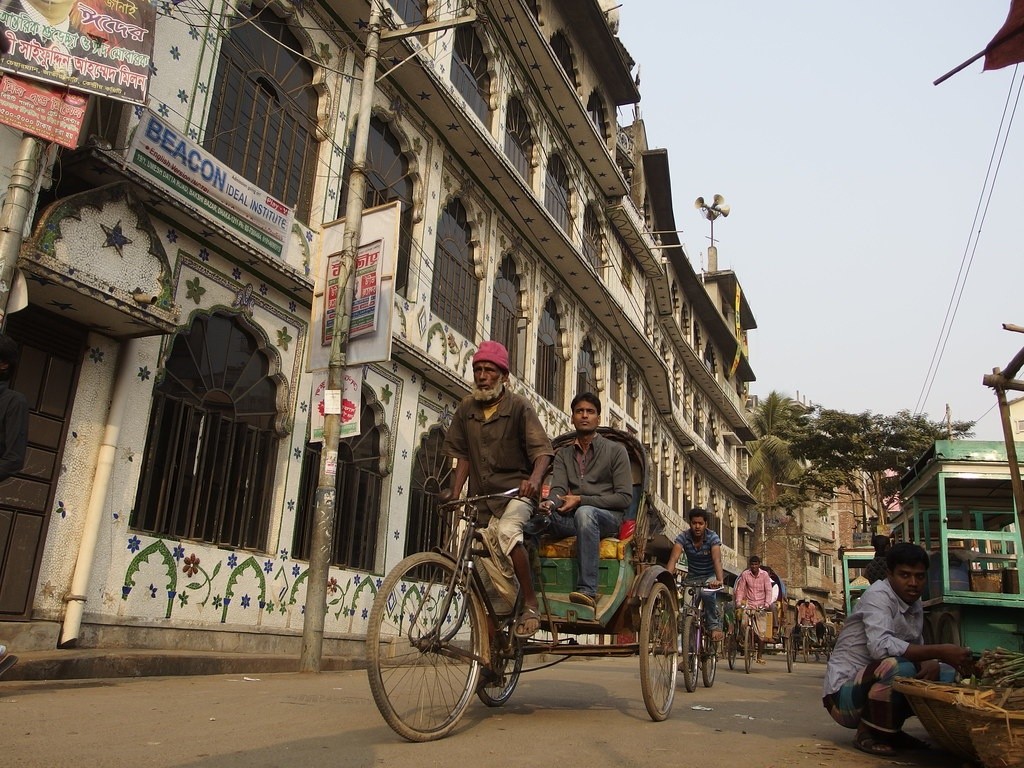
[839,441,1024,655]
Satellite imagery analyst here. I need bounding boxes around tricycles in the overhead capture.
[728,565,795,675]
[790,598,835,663]
[364,426,681,742]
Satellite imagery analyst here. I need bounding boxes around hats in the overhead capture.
[474,342,511,370]
[805,595,811,602]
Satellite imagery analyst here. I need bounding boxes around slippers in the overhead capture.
[891,731,931,748]
[852,733,897,757]
[513,604,542,638]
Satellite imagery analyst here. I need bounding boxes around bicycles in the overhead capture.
[674,575,725,693]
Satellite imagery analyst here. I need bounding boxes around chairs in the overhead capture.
[734,564,785,617]
[533,426,649,559]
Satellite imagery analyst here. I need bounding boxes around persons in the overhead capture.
[735,555,772,665]
[822,543,973,757]
[863,535,891,585]
[524,392,633,607]
[0,0,119,86]
[440,340,555,692]
[799,596,817,629]
[668,509,725,671]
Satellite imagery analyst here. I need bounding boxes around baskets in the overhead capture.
[893,676,1024,768]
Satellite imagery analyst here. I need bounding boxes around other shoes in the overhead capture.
[569,592,594,606]
[738,638,745,647]
[757,659,767,665]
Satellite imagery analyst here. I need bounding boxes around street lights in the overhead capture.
[775,482,860,577]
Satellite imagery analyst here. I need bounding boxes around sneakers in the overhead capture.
[710,629,724,642]
[677,662,686,672]
